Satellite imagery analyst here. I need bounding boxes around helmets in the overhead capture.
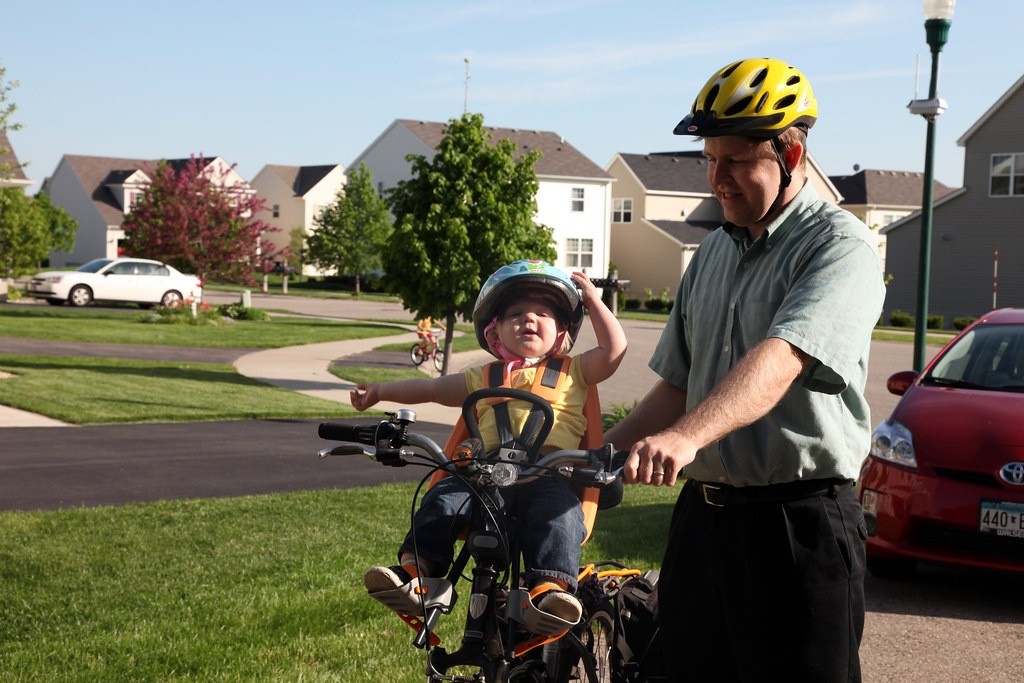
[471,258,584,360]
[671,57,818,142]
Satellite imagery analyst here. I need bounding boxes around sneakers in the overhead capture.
[527,579,582,636]
[364,563,431,616]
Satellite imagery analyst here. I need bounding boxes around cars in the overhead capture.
[854,304,1023,587]
[26,256,204,314]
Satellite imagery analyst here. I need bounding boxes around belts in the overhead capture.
[687,476,852,508]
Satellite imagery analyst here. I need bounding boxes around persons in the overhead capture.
[606,54,895,683]
[415,316,447,360]
[350,258,631,637]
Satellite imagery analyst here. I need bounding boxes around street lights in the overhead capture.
[900,2,957,380]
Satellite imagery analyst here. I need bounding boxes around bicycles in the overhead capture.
[410,328,445,374]
[315,388,691,683]
